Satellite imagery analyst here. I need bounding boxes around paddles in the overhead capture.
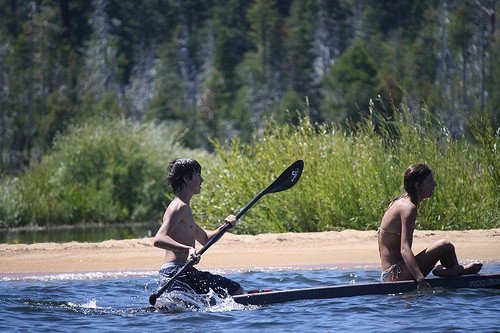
[149,158,305,306]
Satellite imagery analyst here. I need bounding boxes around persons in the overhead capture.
[153,158,246,300]
[378,162,485,295]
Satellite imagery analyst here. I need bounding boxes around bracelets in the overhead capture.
[416,279,426,283]
